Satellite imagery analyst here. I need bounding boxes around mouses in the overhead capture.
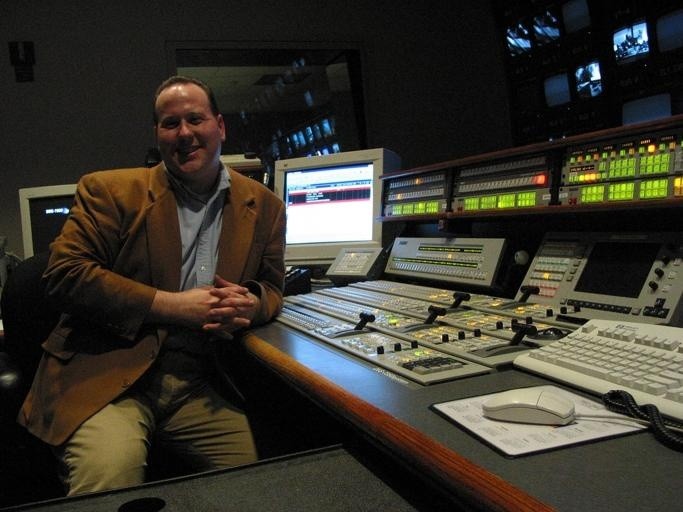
[481,387,575,426]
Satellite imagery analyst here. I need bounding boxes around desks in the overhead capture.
[0,442,416,511]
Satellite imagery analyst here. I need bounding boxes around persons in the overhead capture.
[17,74,288,498]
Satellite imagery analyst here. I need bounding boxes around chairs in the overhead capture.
[6,253,62,380]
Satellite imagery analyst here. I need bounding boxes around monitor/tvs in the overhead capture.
[499,1,683,132]
[18,184,77,260]
[240,58,341,173]
[272,147,410,267]
